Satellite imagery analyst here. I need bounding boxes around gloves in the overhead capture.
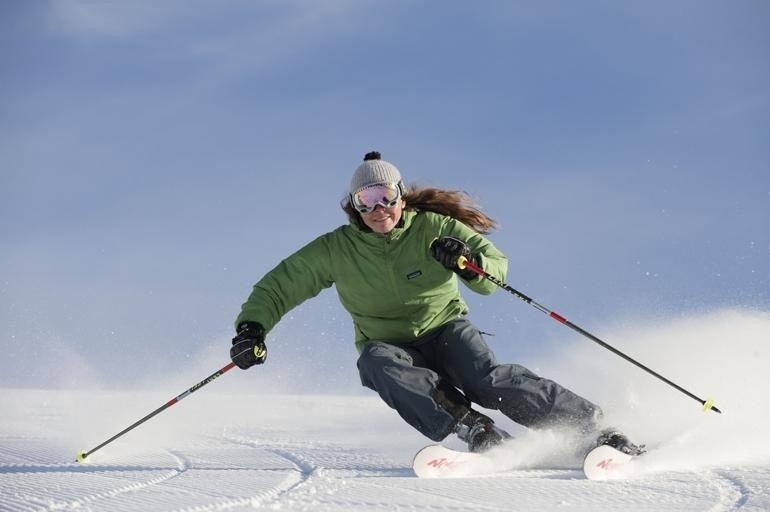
[230,322,268,370]
[429,237,479,282]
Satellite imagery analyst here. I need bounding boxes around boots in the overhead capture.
[455,409,513,454]
[595,427,634,451]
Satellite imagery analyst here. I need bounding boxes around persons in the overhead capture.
[232,151,646,457]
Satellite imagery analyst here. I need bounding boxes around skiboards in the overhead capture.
[412,420,694,480]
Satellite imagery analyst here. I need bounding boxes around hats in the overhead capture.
[351,151,405,206]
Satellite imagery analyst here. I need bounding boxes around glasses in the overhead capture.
[351,181,402,216]
